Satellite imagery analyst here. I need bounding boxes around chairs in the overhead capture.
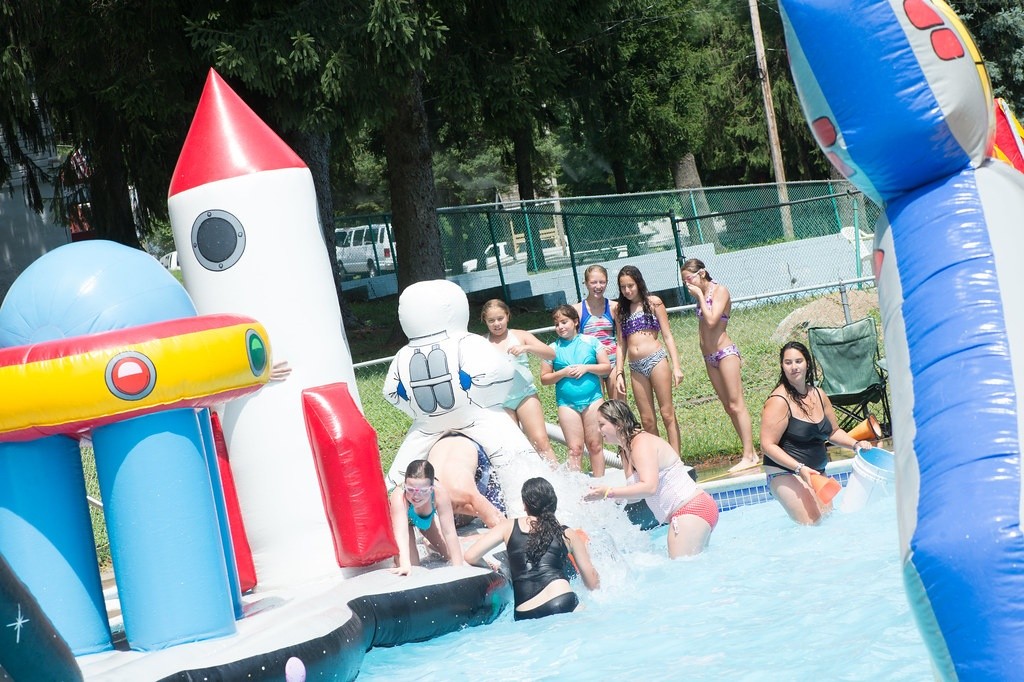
[808,317,894,440]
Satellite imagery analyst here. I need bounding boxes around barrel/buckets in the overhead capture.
[843,448,894,513]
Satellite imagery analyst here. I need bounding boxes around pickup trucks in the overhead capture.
[462,235,569,272]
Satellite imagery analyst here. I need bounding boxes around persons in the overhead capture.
[389,461,462,576]
[585,399,719,558]
[384,279,542,490]
[615,265,683,458]
[539,303,612,477]
[682,259,759,474]
[572,266,627,403]
[431,437,506,527]
[482,299,557,469]
[464,477,598,621]
[759,342,872,526]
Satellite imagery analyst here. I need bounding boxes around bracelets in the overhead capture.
[616,370,623,372]
[616,373,623,380]
[795,463,803,476]
[604,487,611,500]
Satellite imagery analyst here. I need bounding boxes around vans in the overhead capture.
[330,223,401,277]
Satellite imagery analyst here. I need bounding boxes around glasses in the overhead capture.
[681,269,706,283]
[404,484,433,495]
